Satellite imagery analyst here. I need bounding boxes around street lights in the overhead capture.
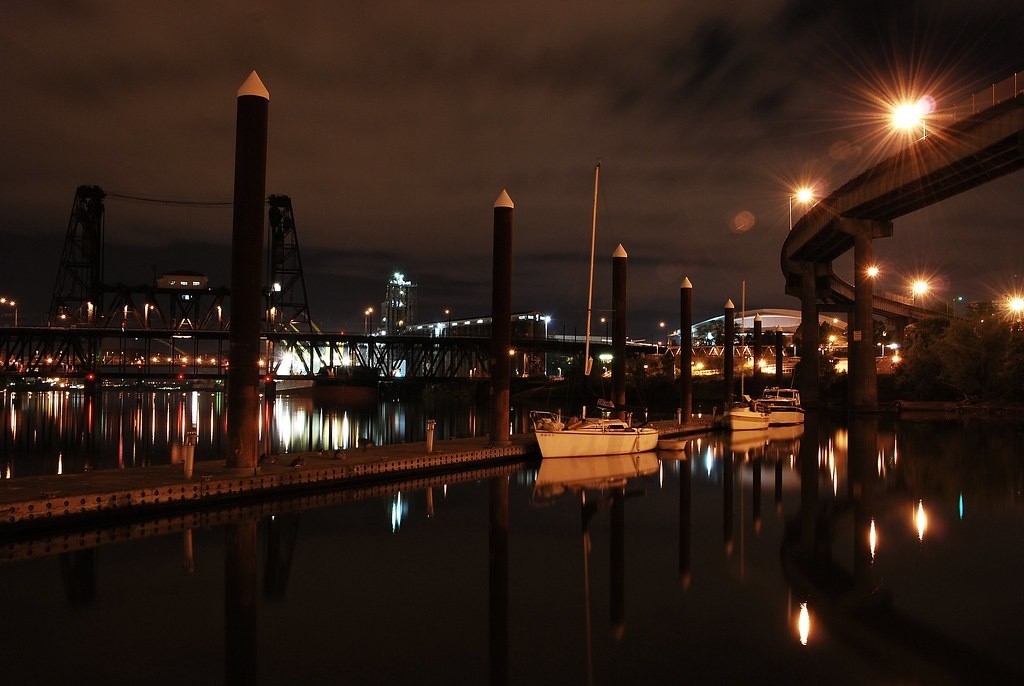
[446,310,451,338]
[365,311,370,335]
[787,188,811,233]
[911,279,930,305]
[368,308,373,335]
[9,302,18,327]
[600,317,610,343]
[544,314,551,340]
[894,106,928,148]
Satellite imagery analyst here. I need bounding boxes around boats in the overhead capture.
[758,386,805,427]
[770,420,810,443]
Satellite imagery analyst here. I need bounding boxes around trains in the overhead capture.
[410,310,547,338]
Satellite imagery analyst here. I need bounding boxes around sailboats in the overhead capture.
[530,159,658,459]
[715,427,772,580]
[535,449,665,686]
[719,279,770,432]
